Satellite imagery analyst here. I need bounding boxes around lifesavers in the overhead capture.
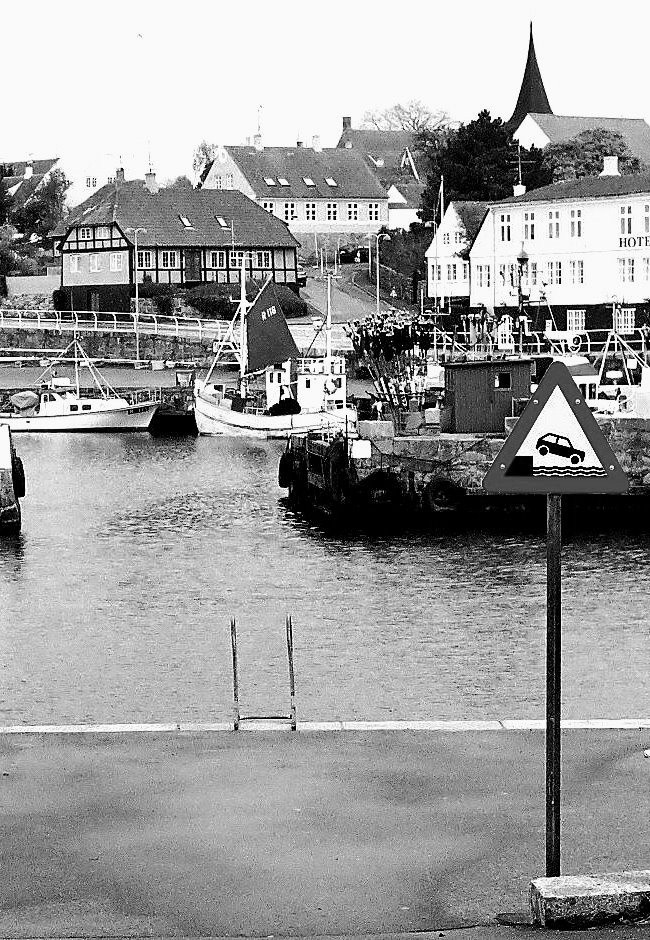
[324,378,338,395]
[361,472,403,516]
[422,477,464,519]
[278,451,298,489]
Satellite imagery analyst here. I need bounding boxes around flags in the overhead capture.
[246,285,301,374]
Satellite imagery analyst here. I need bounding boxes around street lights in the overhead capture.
[513,250,529,321]
[359,234,393,314]
[423,220,440,362]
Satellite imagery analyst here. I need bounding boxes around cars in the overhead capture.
[296,264,309,287]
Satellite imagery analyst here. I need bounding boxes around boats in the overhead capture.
[0,308,163,435]
[188,246,364,442]
